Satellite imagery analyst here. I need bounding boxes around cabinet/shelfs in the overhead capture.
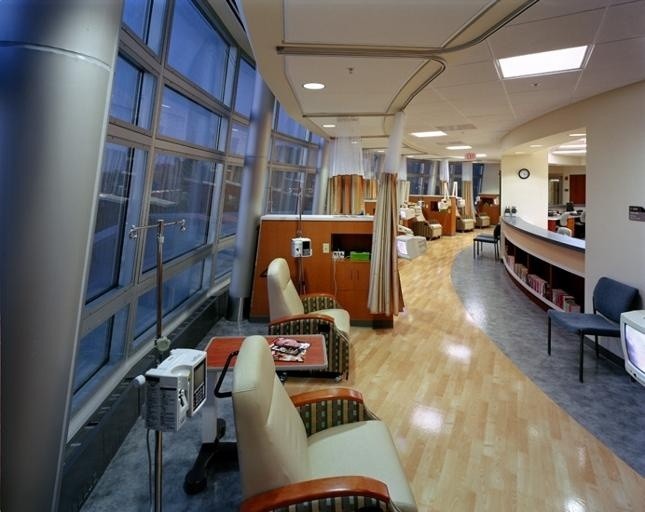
[476,193,500,224]
[247,194,457,329]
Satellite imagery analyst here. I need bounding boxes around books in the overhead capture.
[507,255,581,313]
[268,349,311,363]
[268,336,312,356]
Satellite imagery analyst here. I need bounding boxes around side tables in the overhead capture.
[182,334,329,495]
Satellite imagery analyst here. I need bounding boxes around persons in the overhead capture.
[275,338,310,350]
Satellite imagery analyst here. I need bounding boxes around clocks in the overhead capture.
[518,168,530,179]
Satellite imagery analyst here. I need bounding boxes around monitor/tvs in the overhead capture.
[619,310,645,388]
[400,208,415,220]
[397,235,427,259]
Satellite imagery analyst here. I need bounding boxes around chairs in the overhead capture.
[548,210,585,237]
[260,257,350,382]
[546,277,639,384]
[214,335,419,512]
[455,206,501,261]
